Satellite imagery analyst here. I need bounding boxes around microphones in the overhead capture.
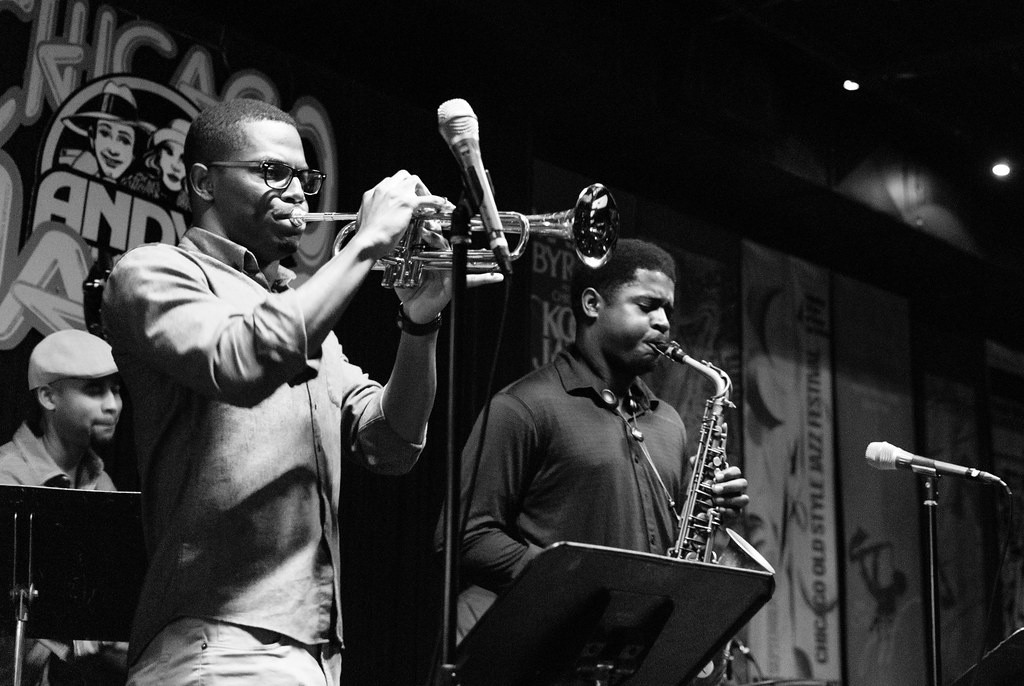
[437,98,518,276]
[864,437,1002,492]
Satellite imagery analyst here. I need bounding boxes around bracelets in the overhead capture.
[397,302,442,336]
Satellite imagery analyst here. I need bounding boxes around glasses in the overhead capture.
[204,161,326,196]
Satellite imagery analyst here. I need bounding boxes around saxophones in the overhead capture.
[648,336,776,685]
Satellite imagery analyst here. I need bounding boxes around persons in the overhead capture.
[0,329,122,490]
[437,239,749,686]
[104,99,453,686]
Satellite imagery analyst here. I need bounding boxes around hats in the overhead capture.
[27,329,118,390]
